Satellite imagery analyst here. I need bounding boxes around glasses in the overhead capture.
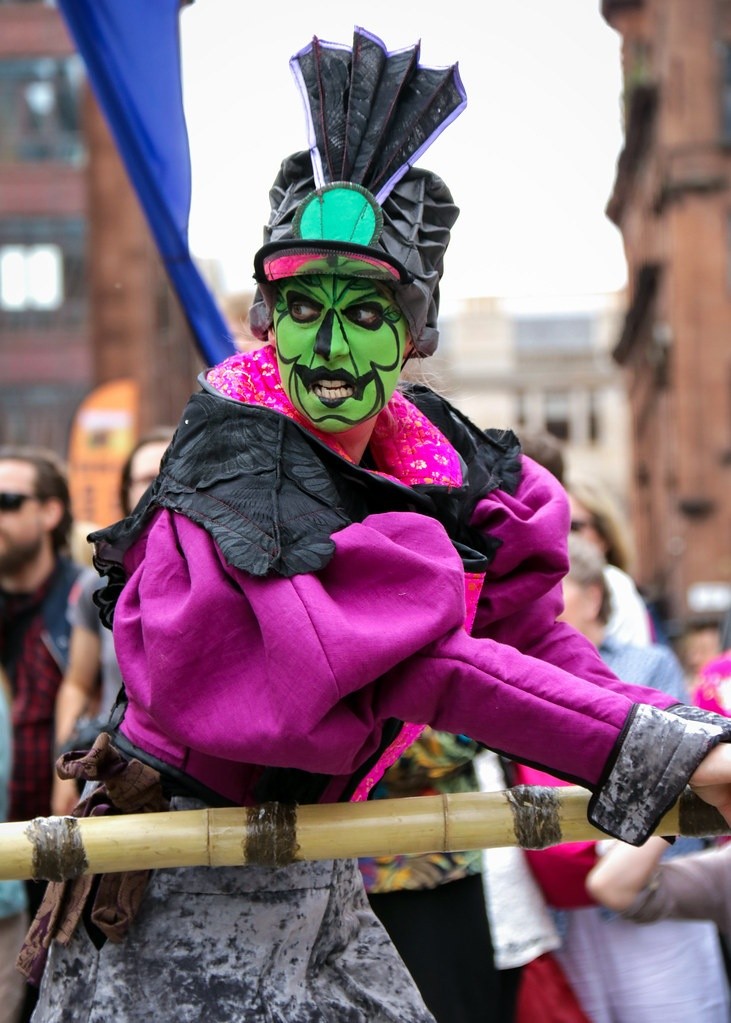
[0,491,43,513]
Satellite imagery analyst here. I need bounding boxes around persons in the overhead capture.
[17,27,731,1023]
[0,428,731,1023]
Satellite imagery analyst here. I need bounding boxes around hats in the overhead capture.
[248,29,460,360]
